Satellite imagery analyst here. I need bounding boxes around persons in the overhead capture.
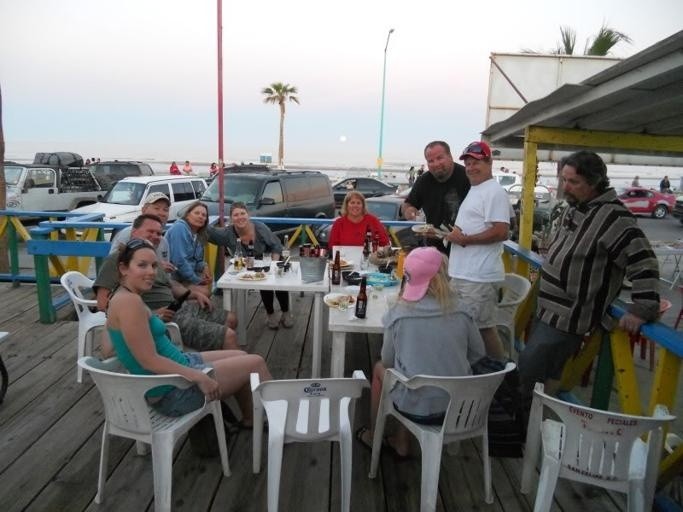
[85,158,91,164]
[357,245,486,460]
[183,161,193,176]
[401,139,471,260]
[92,215,239,351]
[512,150,661,430]
[328,191,389,251]
[202,201,295,329]
[208,163,217,176]
[91,158,95,163]
[170,161,181,174]
[631,175,640,187]
[440,140,516,363]
[164,201,213,296]
[660,176,670,192]
[110,191,177,281]
[97,158,100,162]
[404,166,417,188]
[417,164,424,179]
[102,238,274,428]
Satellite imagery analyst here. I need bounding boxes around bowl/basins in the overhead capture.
[348,278,361,286]
[342,271,359,279]
[277,262,291,273]
[379,266,394,275]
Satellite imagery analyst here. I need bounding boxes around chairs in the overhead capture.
[77,355,233,511]
[520,382,677,512]
[60,270,107,384]
[250,369,370,512]
[496,273,532,361]
[368,362,517,512]
[629,299,672,372]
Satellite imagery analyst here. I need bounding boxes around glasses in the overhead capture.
[462,146,487,159]
[127,238,156,249]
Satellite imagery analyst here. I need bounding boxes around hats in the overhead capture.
[458,141,492,160]
[401,245,442,301]
[143,191,170,204]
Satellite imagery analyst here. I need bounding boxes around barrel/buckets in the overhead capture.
[299,255,329,283]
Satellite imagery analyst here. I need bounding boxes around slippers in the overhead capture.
[383,430,411,463]
[356,425,373,451]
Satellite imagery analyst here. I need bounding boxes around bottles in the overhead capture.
[354,276,367,319]
[246,240,254,270]
[298,245,304,257]
[364,230,373,251]
[304,243,311,257]
[281,235,290,260]
[312,245,319,257]
[332,251,340,285]
[319,247,327,257]
[363,239,370,257]
[371,240,378,255]
[234,239,242,270]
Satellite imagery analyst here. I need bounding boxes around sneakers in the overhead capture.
[267,311,280,328]
[280,311,294,329]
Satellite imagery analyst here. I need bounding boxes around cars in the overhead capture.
[321,196,406,241]
[618,187,675,219]
[332,176,399,205]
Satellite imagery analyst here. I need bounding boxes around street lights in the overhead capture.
[377,28,395,178]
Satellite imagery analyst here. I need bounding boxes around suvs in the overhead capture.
[3,164,107,212]
[61,175,208,240]
[175,165,336,231]
[87,161,155,193]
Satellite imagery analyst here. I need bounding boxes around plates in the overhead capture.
[412,224,444,239]
[363,272,400,287]
[237,272,267,281]
[323,293,358,309]
[329,260,354,269]
[230,258,247,265]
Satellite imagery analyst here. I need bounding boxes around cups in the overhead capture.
[263,252,272,266]
[337,295,351,311]
[361,255,369,269]
[275,267,284,278]
